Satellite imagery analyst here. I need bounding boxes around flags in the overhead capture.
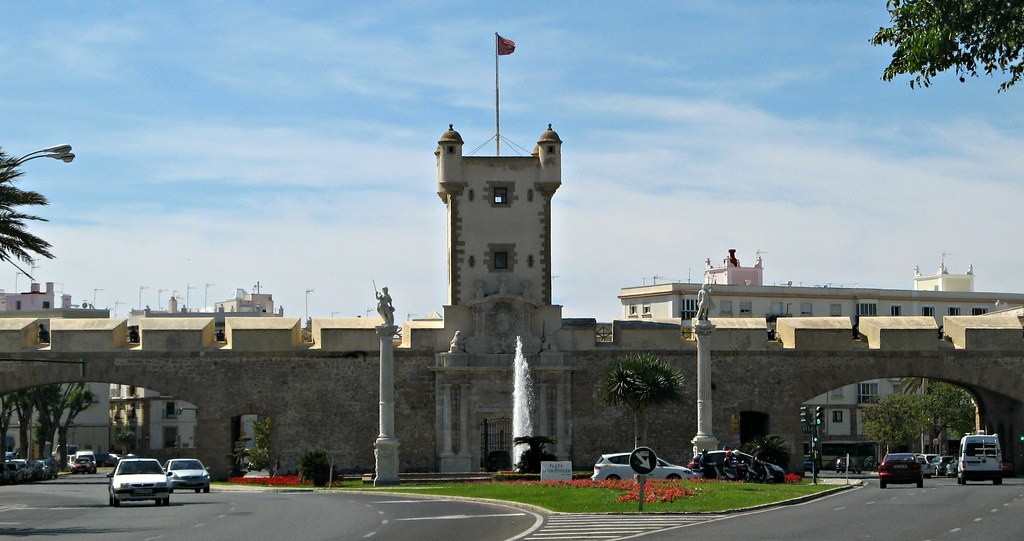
[498,36,515,55]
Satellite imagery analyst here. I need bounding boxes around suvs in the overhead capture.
[880,453,923,488]
[687,451,785,487]
[835,458,861,474]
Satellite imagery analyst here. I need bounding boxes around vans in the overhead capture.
[51,445,79,456]
[75,450,97,473]
[957,429,1004,485]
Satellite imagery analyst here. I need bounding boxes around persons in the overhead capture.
[938,326,943,339]
[852,323,860,339]
[695,284,712,321]
[38,323,49,343]
[273,460,280,477]
[217,330,224,341]
[699,445,764,482]
[129,325,140,342]
[768,329,774,340]
[376,287,395,323]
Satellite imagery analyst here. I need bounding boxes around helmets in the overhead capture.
[726,450,732,457]
[733,449,740,456]
[756,446,763,450]
[702,449,708,453]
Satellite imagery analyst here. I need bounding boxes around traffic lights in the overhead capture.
[800,406,807,422]
[813,430,818,442]
[816,406,823,426]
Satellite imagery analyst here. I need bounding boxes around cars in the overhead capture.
[590,451,695,483]
[915,451,958,479]
[804,454,820,473]
[67,455,76,471]
[75,457,95,474]
[0,451,60,487]
[163,458,211,493]
[107,457,170,507]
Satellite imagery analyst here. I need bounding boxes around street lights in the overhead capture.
[90,279,315,322]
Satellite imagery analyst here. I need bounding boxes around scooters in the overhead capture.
[691,461,776,484]
